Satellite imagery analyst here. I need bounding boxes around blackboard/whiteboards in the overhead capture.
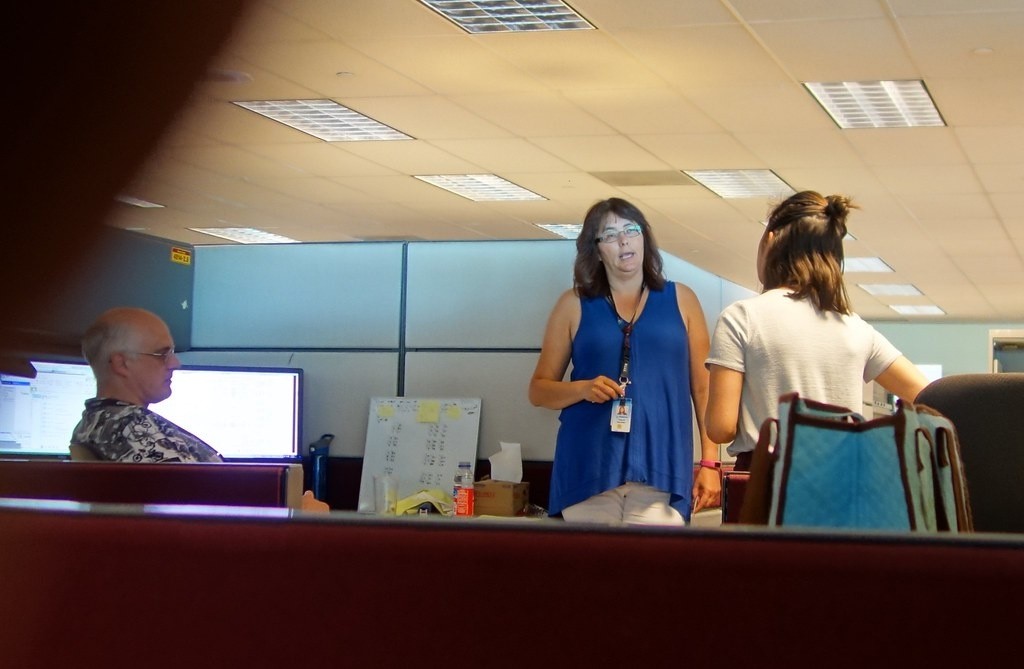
[357,396,481,517]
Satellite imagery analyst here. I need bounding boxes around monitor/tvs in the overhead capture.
[0,354,97,459]
[148,364,303,463]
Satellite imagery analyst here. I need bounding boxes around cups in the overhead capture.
[373,473,398,516]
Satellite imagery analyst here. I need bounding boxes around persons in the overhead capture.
[528,197,722,519]
[704,191,931,471]
[71,306,329,515]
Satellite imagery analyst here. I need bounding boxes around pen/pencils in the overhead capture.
[591,382,631,404]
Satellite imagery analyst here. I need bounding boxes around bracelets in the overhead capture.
[700,460,722,471]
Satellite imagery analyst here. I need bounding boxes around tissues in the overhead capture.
[471,442,530,516]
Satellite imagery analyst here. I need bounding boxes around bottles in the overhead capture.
[452,461,475,516]
[308,432,335,502]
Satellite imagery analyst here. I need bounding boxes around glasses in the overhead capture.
[594,223,642,241]
[135,345,176,358]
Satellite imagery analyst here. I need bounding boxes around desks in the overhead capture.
[0,497,1024,669]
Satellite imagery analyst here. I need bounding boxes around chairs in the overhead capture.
[916,371,1024,534]
